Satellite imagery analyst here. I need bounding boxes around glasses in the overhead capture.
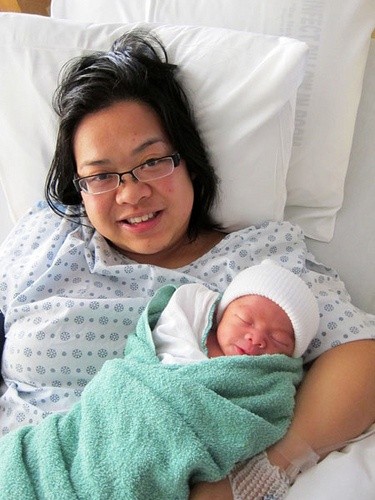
[72,151,181,195]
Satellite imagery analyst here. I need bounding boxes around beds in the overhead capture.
[0,1,375,498]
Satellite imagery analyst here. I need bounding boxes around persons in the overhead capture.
[0,27,374,500]
[0,258,322,500]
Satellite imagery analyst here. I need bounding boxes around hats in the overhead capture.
[216,260,319,358]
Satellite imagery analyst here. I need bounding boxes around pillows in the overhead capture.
[50,0,375,243]
[0,13,307,233]
